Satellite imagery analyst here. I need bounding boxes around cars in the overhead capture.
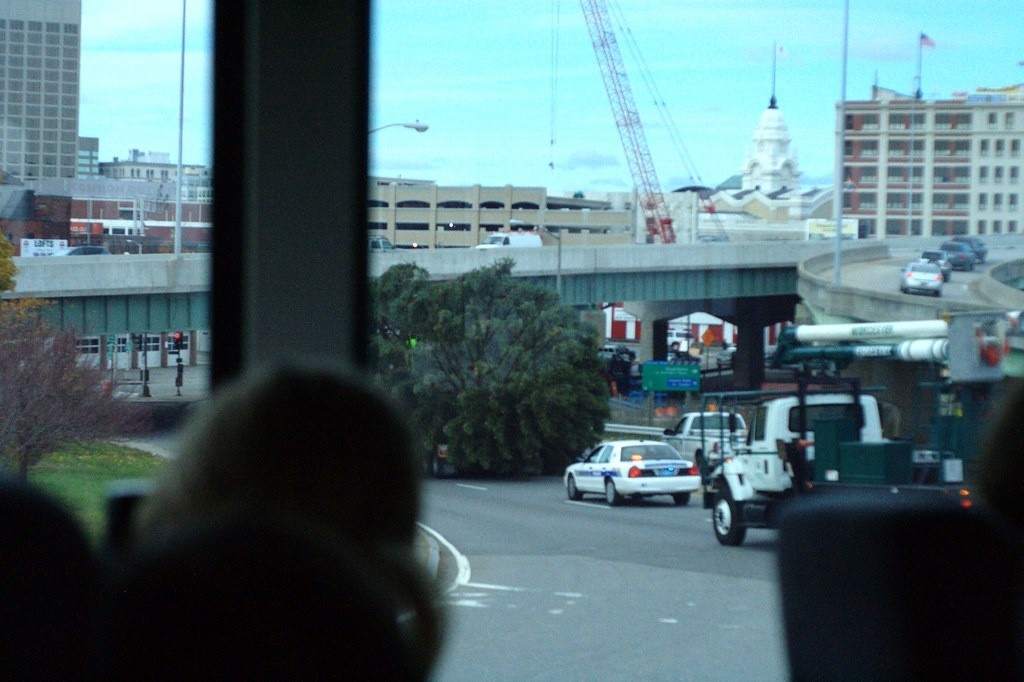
[589,315,1024,549]
[51,244,113,256]
[953,234,989,263]
[920,249,954,283]
[900,260,944,297]
[941,240,977,272]
[564,439,704,507]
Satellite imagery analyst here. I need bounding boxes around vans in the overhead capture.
[476,229,543,252]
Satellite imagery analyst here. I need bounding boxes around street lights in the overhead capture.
[127,239,143,253]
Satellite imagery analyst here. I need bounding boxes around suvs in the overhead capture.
[366,234,394,253]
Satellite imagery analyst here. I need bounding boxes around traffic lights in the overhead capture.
[173,331,182,347]
[133,332,142,351]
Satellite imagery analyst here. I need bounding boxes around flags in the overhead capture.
[774,41,787,58]
[920,32,936,47]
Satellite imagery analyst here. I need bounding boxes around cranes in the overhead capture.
[542,1,730,245]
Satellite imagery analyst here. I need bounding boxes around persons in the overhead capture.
[917,385,1024,682]
[0,359,447,682]
